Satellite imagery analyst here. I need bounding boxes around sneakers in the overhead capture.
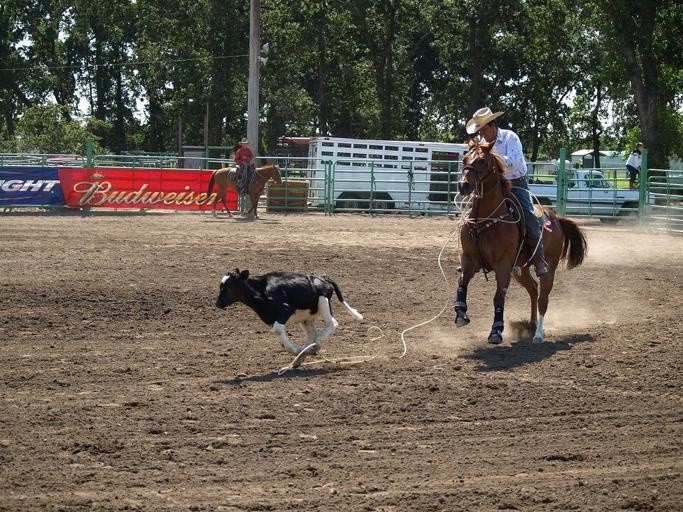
[534,255,548,277]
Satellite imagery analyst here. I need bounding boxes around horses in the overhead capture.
[206,164,282,220]
[455,135,589,344]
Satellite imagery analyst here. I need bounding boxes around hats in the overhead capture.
[238,137,250,146]
[465,107,506,135]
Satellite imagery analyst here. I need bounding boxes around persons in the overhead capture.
[462,106,548,277]
[233,138,255,196]
[624,141,643,189]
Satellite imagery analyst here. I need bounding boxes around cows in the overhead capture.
[215,268,364,368]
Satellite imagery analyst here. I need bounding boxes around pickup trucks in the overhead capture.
[526,162,656,227]
